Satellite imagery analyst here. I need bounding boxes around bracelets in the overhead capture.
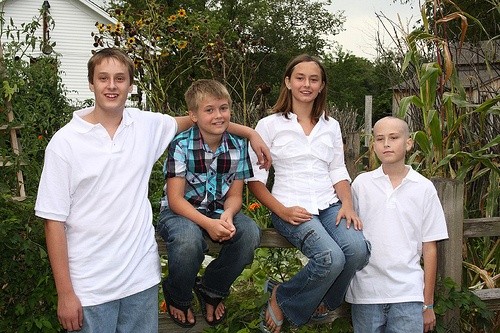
[422,305,433,312]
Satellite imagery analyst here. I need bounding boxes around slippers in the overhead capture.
[310,300,329,320]
[162,282,197,328]
[259,279,284,333]
[193,277,226,326]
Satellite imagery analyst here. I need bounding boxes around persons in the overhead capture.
[243,55,370,333]
[345,116,448,333]
[159,79,260,328]
[34,48,272,333]
